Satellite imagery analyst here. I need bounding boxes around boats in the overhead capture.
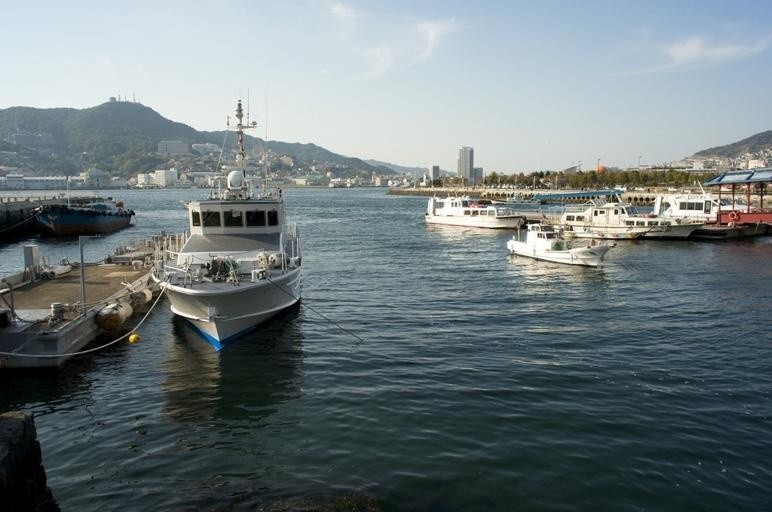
[29,172,136,237]
[147,93,306,352]
[503,256,620,293]
[505,210,617,270]
[169,315,289,376]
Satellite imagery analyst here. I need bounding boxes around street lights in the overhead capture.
[597,158,601,186]
[638,156,643,184]
